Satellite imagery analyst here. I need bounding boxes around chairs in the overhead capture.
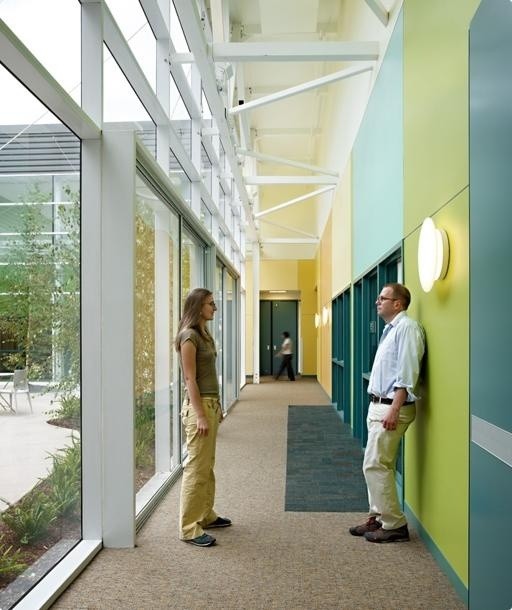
[0,366,33,415]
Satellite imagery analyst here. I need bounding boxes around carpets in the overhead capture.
[284,405,370,512]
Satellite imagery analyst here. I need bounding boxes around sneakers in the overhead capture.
[365,524,409,542]
[349,517,382,535]
[208,517,231,528]
[187,533,215,546]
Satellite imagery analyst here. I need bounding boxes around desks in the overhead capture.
[0,372,15,415]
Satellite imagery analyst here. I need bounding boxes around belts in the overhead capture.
[369,395,410,406]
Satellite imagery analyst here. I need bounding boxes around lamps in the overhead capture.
[314,313,320,328]
[417,217,450,292]
[322,307,329,325]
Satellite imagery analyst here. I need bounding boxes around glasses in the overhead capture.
[377,296,393,301]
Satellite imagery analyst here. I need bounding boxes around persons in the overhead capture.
[348,283,426,544]
[275,330,295,381]
[175,287,232,549]
